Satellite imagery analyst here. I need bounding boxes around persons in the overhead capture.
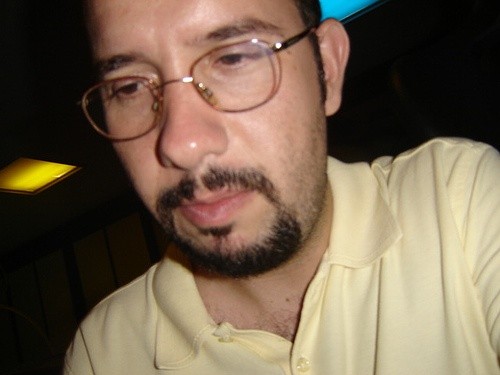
[60,0,500,375]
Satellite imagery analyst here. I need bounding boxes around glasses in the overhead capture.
[76,21,317,143]
